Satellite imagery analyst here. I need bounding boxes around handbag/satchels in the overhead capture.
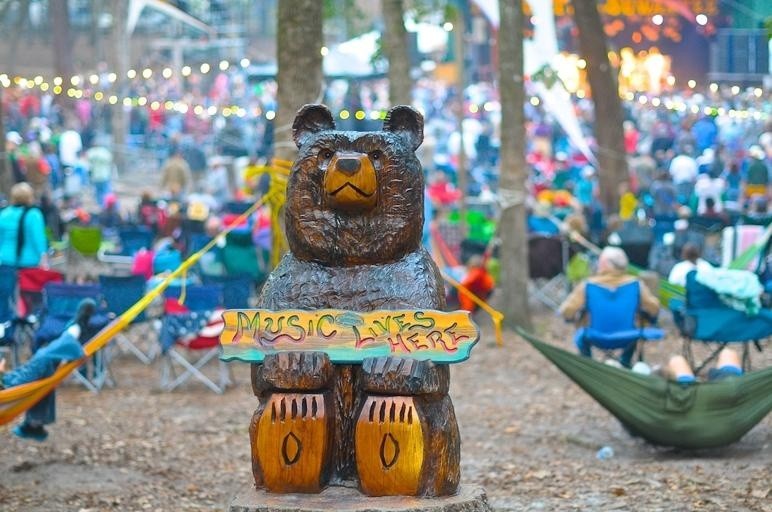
[13,267,66,320]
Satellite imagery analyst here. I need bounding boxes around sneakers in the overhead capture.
[0,349,33,374]
[13,421,49,442]
[64,297,96,343]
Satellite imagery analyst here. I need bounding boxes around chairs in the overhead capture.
[0,209,269,396]
[449,181,769,375]
